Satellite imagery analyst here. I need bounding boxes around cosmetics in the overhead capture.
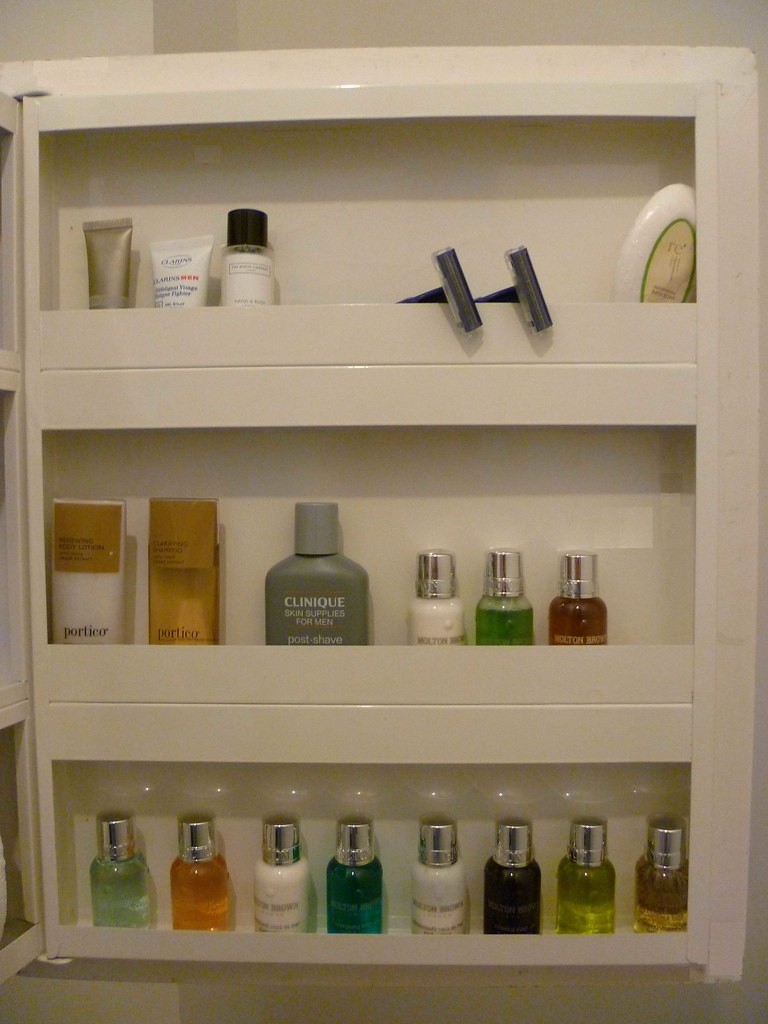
[264,500,370,648]
[474,548,535,647]
[51,498,128,646]
[407,549,468,647]
[89,809,152,932]
[82,217,133,310]
[631,810,689,934]
[149,234,217,308]
[147,495,220,646]
[168,807,231,932]
[253,809,310,935]
[326,810,383,936]
[547,549,608,646]
[608,181,698,306]
[481,816,542,935]
[410,810,472,936]
[219,207,275,306]
[554,814,617,936]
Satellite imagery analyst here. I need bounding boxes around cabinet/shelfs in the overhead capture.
[12,44,768,991]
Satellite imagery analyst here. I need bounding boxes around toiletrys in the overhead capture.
[612,182,699,306]
[79,206,276,308]
[50,490,610,643]
[87,809,693,937]
